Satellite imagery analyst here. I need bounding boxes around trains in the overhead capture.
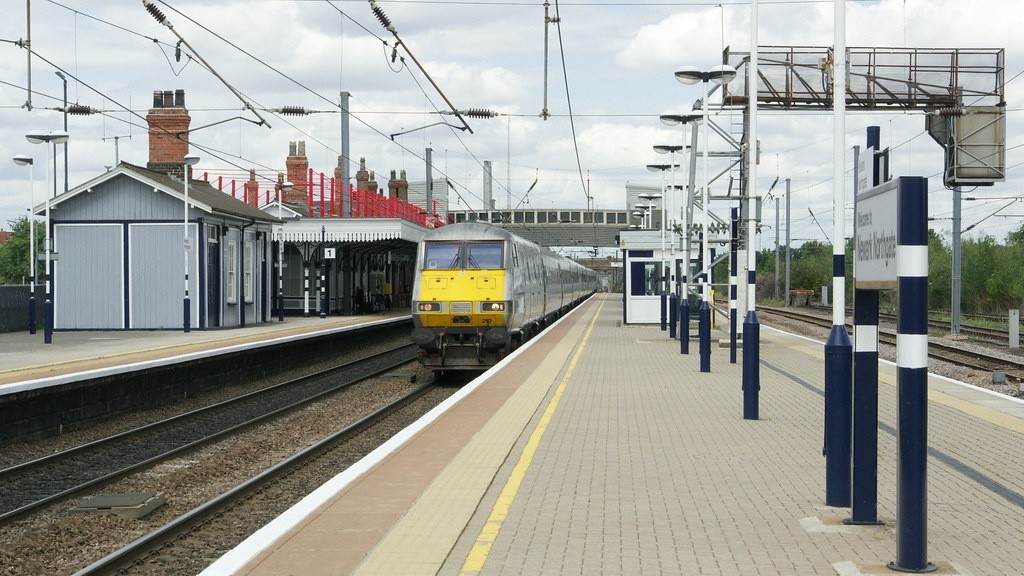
[408,219,599,381]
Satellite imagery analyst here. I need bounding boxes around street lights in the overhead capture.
[12,153,36,334]
[182,153,201,333]
[652,141,691,354]
[23,129,70,343]
[659,109,709,354]
[673,64,737,372]
[632,162,682,331]
[276,181,295,322]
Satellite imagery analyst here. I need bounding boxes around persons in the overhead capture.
[356,286,368,314]
